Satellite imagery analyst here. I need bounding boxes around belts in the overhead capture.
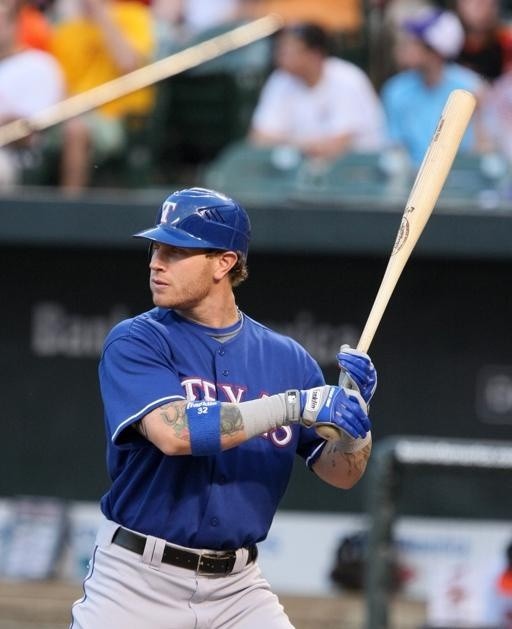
[112,526,258,576]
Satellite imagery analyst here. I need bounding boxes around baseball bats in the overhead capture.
[316,89,476,443]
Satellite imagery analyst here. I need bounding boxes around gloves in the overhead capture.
[277,385,373,441]
[335,344,378,406]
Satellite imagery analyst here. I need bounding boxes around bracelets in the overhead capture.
[235,392,289,439]
[331,429,373,452]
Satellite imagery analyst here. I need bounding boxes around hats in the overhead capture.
[401,6,466,59]
[129,187,252,266]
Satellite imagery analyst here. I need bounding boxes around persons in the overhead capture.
[66,183,378,629]
[1,2,510,204]
